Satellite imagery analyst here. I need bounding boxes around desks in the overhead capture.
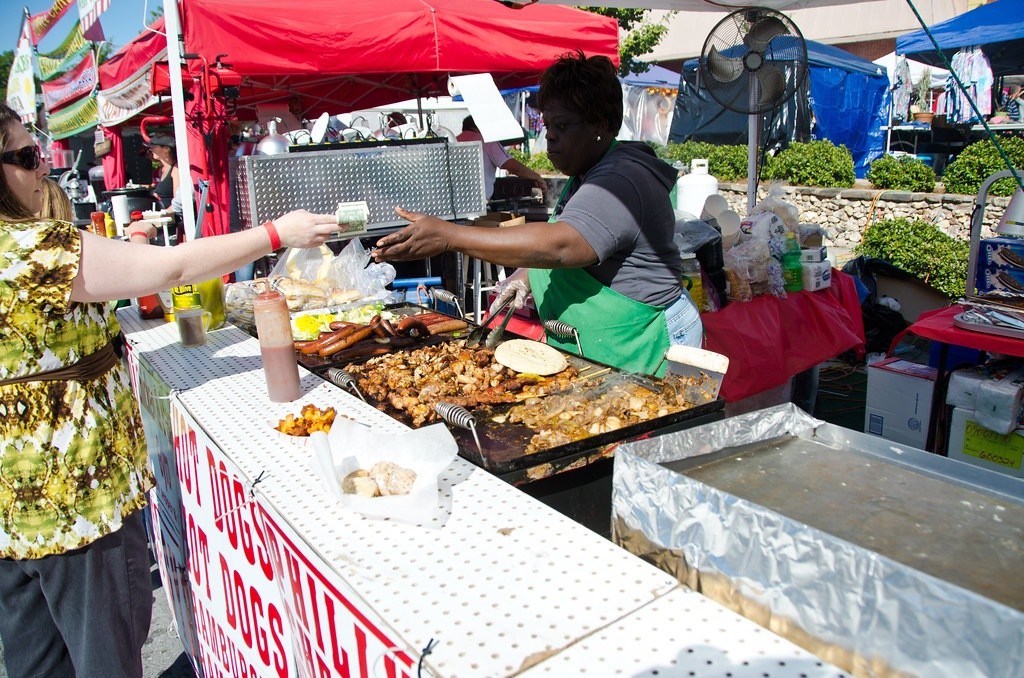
[482,267,865,418]
[911,303,1024,456]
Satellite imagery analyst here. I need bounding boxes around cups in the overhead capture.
[176,308,212,347]
[700,194,741,250]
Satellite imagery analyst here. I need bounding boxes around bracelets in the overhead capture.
[263,221,281,252]
[131,232,147,238]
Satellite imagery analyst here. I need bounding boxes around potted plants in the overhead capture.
[914,67,935,125]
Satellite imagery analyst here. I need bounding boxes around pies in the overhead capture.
[494,339,568,376]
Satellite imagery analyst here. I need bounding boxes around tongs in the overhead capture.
[466,292,516,347]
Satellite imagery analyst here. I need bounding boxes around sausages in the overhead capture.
[294,313,470,357]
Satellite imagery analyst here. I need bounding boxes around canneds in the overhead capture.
[171,276,227,330]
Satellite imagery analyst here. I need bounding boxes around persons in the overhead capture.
[456,115,548,214]
[371,48,704,379]
[142,131,182,239]
[0,103,350,678]
[34,178,157,244]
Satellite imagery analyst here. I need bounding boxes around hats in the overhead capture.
[143,130,176,149]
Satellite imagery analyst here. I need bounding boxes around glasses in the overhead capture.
[0,143,43,171]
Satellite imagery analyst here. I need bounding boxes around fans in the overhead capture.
[700,7,808,214]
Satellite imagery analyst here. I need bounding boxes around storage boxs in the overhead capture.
[473,213,525,228]
[865,357,937,451]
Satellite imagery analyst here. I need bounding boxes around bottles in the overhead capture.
[137,276,226,331]
[783,232,803,292]
[679,252,702,312]
[253,279,301,402]
[91,211,117,239]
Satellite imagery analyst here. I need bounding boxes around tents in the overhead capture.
[667,36,891,179]
[452,85,540,158]
[95,0,621,243]
[531,58,681,155]
[886,0,1024,154]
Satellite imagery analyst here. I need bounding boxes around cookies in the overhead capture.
[996,272,1024,294]
[997,248,1024,269]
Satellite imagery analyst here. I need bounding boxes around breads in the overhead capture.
[236,274,361,311]
[340,462,416,497]
